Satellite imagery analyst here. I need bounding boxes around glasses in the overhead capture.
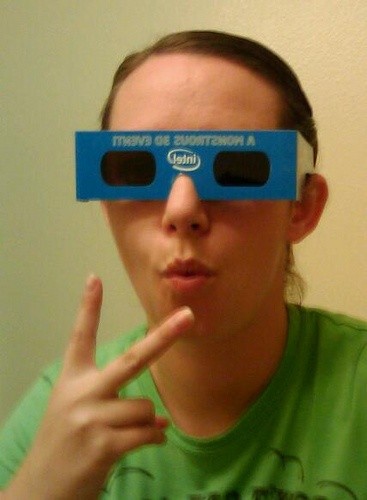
[75,130,314,202]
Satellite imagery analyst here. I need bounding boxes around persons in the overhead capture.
[0,28,367,500]
